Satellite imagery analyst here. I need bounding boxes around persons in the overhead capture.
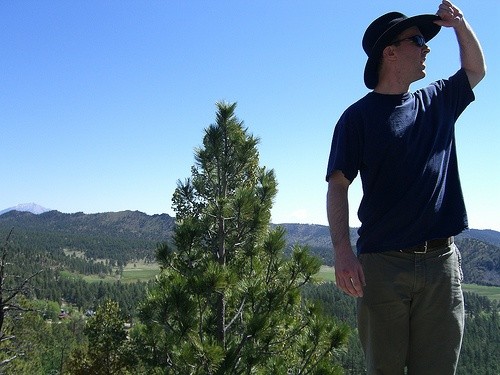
[326,0,487,375]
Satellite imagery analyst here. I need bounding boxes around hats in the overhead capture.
[362,11,442,89]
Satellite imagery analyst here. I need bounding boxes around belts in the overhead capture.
[401,236,455,255]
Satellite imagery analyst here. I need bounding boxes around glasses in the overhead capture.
[388,35,425,47]
